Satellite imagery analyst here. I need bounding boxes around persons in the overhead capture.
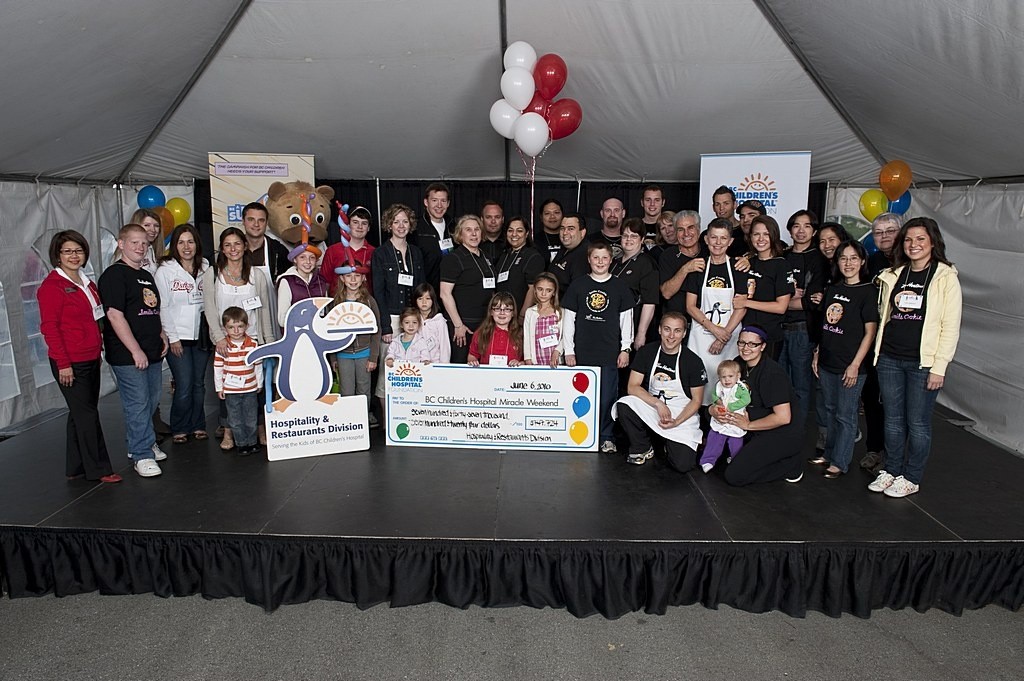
[214,307,264,457]
[610,311,709,474]
[202,183,907,478]
[97,210,172,477]
[154,223,210,443]
[331,258,382,428]
[36,229,123,483]
[560,241,635,453]
[811,239,880,478]
[722,326,808,486]
[437,214,497,364]
[868,216,962,497]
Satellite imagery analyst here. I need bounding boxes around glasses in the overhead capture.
[491,307,514,313]
[873,229,900,236]
[840,256,860,263]
[59,248,86,255]
[737,340,763,348]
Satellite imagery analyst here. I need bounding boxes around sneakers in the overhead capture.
[600,441,617,453]
[128,442,167,460]
[627,445,655,465]
[134,457,162,477]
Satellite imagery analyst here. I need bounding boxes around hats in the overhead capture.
[735,200,766,215]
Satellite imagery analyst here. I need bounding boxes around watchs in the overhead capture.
[622,348,631,354]
[812,348,820,355]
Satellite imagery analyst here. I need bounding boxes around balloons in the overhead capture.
[335,200,369,275]
[858,160,913,223]
[488,39,582,160]
[137,185,192,238]
[288,191,322,262]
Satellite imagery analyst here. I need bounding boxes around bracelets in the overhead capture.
[454,324,464,329]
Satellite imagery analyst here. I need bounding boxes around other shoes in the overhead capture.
[155,420,171,433]
[156,434,163,440]
[192,430,209,440]
[220,438,234,449]
[701,428,919,498]
[173,434,187,443]
[249,443,260,452]
[66,473,87,480]
[237,445,250,455]
[215,428,225,437]
[100,473,123,483]
[368,410,379,428]
[259,432,266,445]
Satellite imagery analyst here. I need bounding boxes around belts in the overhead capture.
[782,323,807,331]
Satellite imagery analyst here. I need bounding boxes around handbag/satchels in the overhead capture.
[331,368,340,394]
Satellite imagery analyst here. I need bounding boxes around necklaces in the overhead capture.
[602,232,621,244]
[224,266,242,282]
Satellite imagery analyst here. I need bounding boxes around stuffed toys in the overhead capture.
[264,180,334,268]
